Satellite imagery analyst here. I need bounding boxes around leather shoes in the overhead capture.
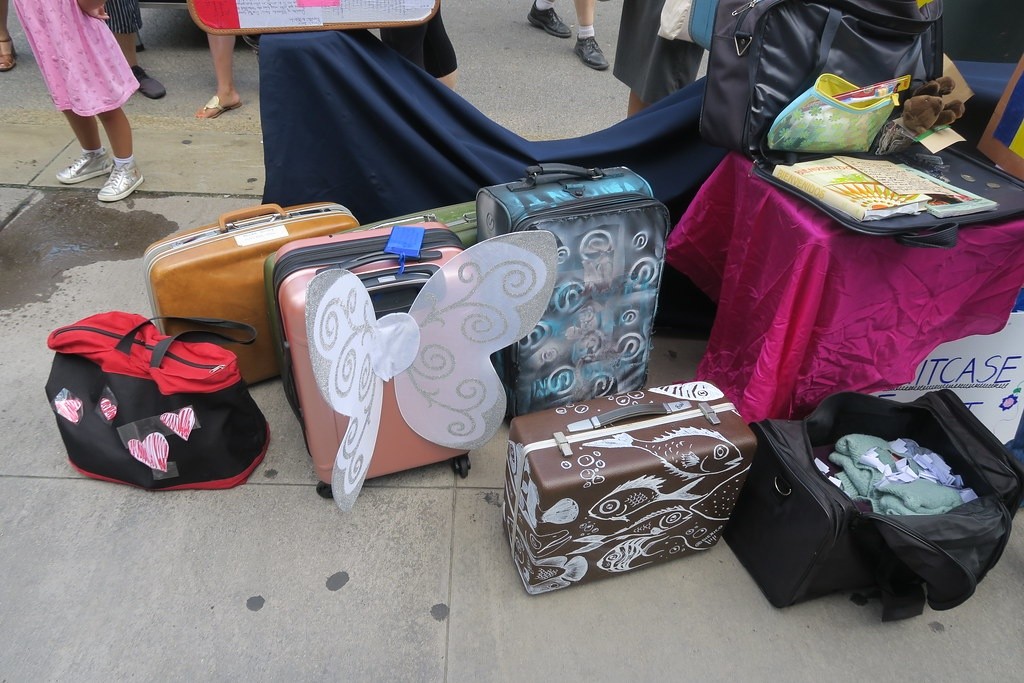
[527,0,571,38]
[573,33,610,70]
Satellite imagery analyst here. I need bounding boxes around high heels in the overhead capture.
[0,35,17,71]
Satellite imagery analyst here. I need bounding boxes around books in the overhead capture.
[771,156,998,221]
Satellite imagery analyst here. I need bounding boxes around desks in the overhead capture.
[666,136,1024,426]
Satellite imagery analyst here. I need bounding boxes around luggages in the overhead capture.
[476,163,670,426]
[271,200,558,498]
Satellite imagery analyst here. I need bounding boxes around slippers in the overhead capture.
[196,96,241,119]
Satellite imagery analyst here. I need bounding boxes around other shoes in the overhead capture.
[131,65,166,99]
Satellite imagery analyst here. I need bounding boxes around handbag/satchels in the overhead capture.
[721,389,1024,623]
[700,0,1024,248]
[44,312,271,492]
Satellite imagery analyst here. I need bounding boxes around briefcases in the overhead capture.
[144,201,359,386]
[503,381,756,594]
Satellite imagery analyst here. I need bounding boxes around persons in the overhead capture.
[527,0,610,69]
[0,0,18,70]
[378,4,459,92]
[196,34,242,118]
[11,0,145,202]
[104,0,166,100]
[612,0,705,118]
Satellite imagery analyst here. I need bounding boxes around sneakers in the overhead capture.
[97,155,144,202]
[57,148,112,184]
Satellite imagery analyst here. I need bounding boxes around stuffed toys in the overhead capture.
[902,76,965,133]
